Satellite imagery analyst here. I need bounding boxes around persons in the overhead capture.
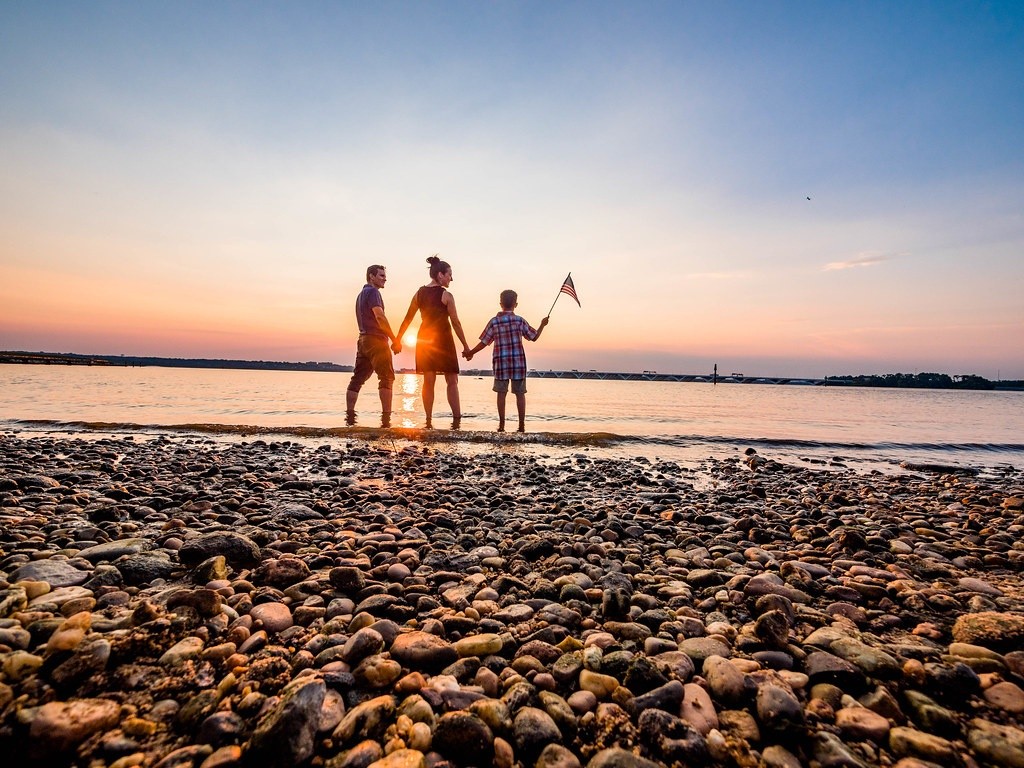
[393,256,473,418]
[346,264,402,412]
[464,290,549,433]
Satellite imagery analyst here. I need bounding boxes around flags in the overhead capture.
[561,276,581,307]
